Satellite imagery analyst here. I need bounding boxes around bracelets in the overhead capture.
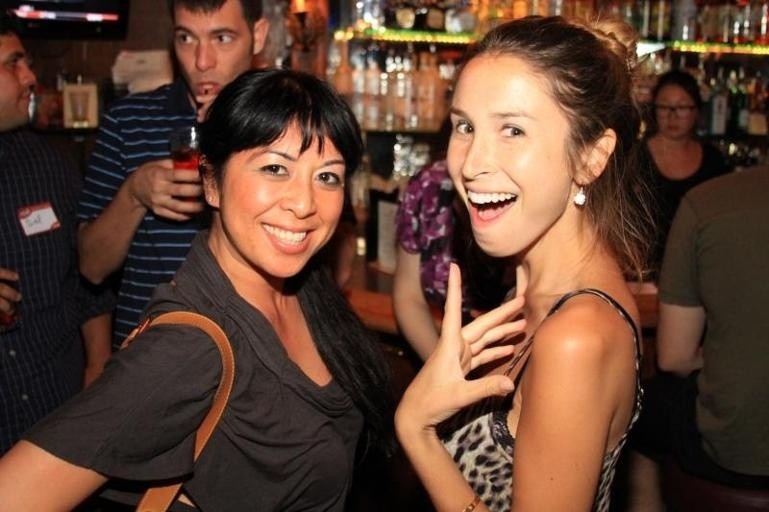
[463,496,481,512]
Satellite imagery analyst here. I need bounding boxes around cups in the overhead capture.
[168,126,209,217]
[63,74,91,128]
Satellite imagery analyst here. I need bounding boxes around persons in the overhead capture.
[0,18,113,456]
[656,164,769,511]
[72,1,268,362]
[391,14,641,512]
[612,63,738,508]
[0,65,397,511]
[392,107,514,367]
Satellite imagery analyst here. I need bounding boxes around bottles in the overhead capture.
[322,0,769,210]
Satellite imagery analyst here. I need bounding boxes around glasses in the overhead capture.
[654,102,695,119]
